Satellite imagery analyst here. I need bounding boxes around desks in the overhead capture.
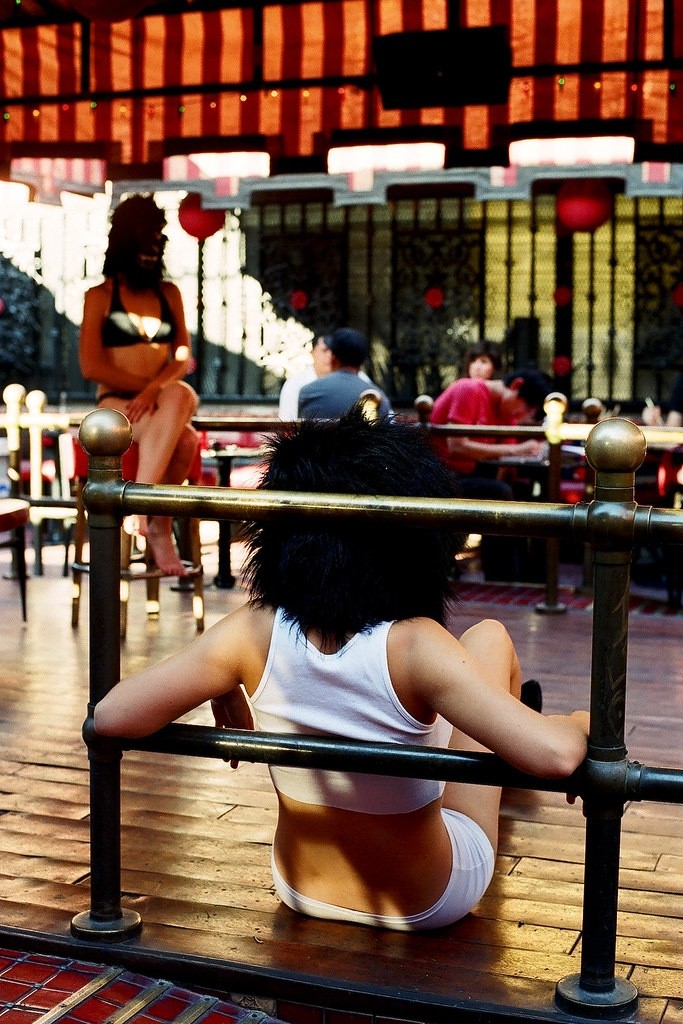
[489,453,583,582]
[197,447,277,588]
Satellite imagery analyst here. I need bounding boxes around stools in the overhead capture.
[0,496,31,621]
[56,433,205,638]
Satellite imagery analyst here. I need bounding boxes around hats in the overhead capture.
[324,328,368,366]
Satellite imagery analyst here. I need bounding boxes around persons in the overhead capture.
[642,397,683,497]
[94,402,590,933]
[79,193,200,574]
[279,328,555,583]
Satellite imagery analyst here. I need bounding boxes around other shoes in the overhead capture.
[520,679,542,713]
